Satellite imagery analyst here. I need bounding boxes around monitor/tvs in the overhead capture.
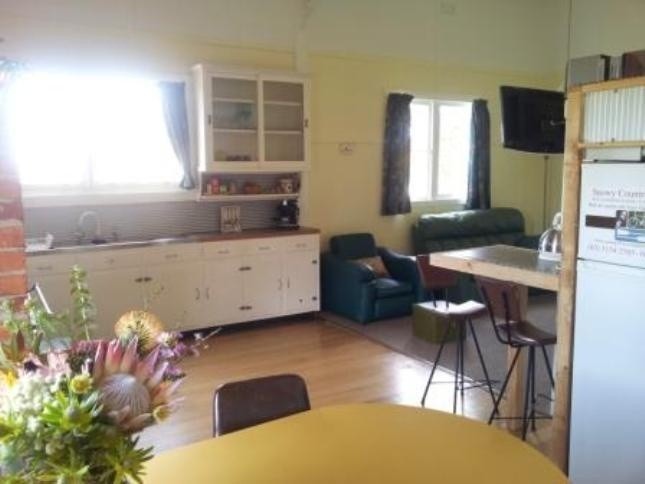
[500,84,565,155]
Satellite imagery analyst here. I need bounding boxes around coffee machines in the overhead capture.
[275,198,300,231]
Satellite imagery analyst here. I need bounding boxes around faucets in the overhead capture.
[74,211,101,244]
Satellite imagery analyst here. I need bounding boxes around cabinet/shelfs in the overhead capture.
[188,61,311,204]
[26,233,320,343]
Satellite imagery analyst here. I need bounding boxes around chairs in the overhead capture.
[321,232,423,323]
[213,374,310,438]
[415,254,557,440]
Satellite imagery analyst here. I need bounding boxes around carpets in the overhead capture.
[321,295,556,400]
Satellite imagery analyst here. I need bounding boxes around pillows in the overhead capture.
[346,255,389,278]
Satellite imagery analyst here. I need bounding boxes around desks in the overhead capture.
[128,402,571,484]
[431,243,559,439]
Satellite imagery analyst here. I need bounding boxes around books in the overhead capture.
[218,203,242,233]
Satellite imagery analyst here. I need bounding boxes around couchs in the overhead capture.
[413,206,552,303]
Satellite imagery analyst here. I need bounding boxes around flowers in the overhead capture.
[1,265,221,484]
